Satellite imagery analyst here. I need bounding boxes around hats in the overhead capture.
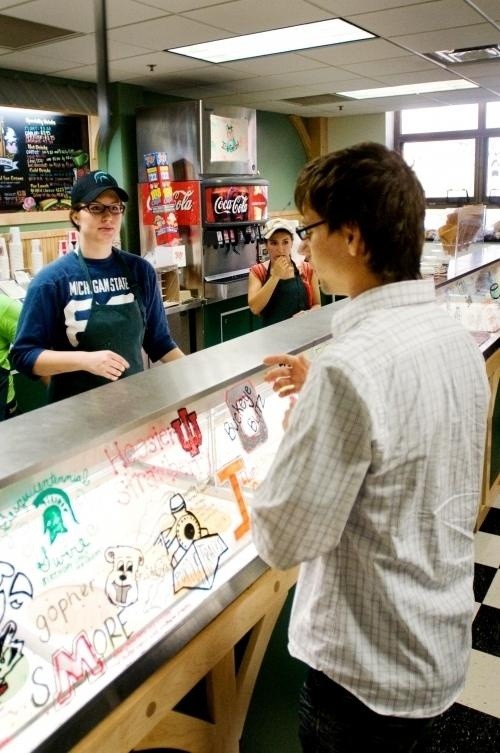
[71,170,128,204]
[263,218,294,240]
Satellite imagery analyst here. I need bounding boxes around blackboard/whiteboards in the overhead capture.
[0,104,90,214]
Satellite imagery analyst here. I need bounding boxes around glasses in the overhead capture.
[79,200,125,215]
[296,221,325,240]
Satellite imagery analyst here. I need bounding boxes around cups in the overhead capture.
[57,230,77,258]
[72,149,90,169]
[0,225,47,281]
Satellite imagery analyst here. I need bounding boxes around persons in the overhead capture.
[250,144,493,753]
[0,244,52,421]
[7,171,186,403]
[247,218,322,330]
